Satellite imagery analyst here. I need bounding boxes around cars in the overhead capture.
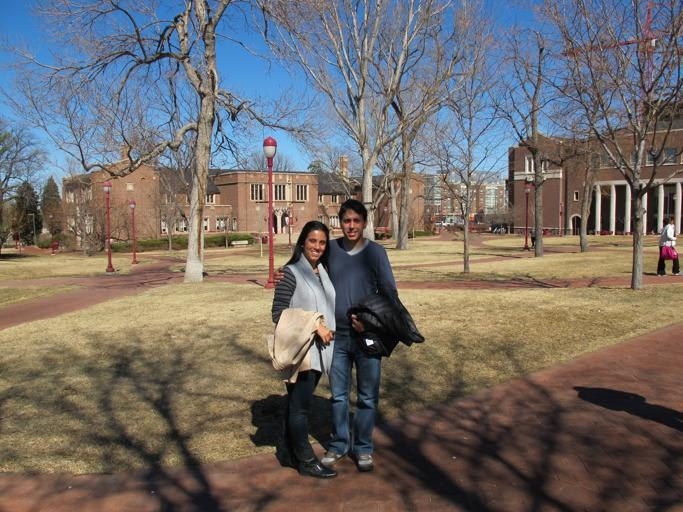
[435,216,506,234]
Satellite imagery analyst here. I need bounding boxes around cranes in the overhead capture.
[556,0,682,123]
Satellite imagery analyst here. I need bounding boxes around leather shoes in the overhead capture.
[298,457,337,478]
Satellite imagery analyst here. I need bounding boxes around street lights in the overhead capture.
[261,135,281,290]
[48,212,56,257]
[128,199,140,265]
[521,180,532,250]
[26,212,37,250]
[101,180,116,272]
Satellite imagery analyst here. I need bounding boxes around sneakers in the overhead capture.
[355,453,374,472]
[320,451,348,466]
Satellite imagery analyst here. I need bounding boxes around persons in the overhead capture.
[270,221,337,478]
[657,217,681,277]
[530,227,536,246]
[491,223,507,235]
[274,199,398,472]
